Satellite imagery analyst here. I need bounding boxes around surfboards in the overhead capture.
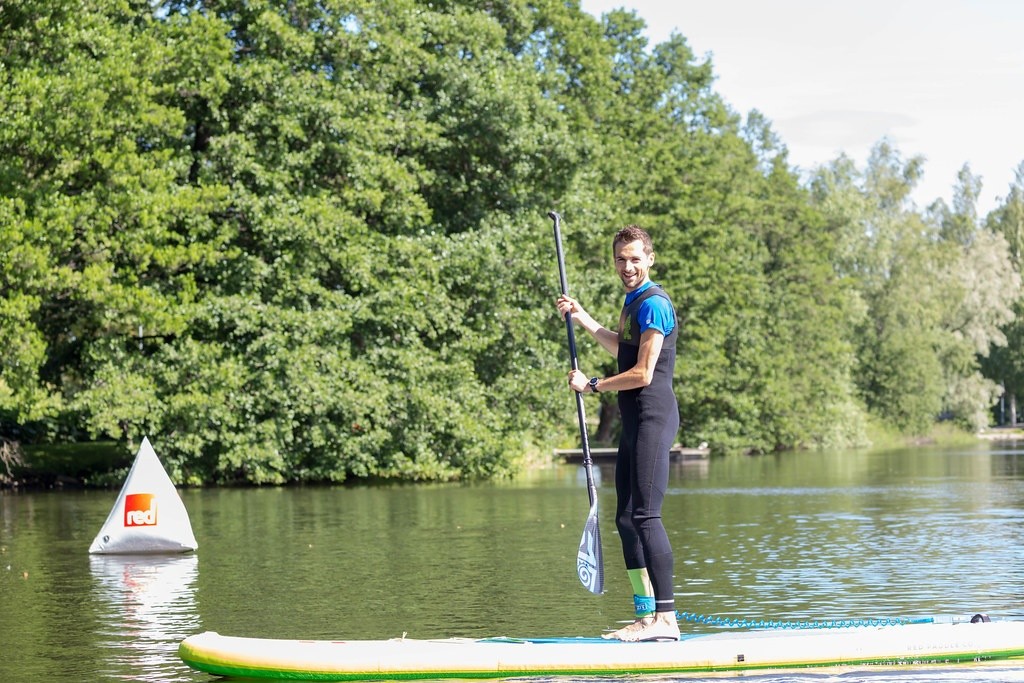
[177,612,1024,680]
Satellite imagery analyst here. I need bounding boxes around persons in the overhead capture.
[556,225,681,642]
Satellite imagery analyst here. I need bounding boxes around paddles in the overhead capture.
[544,209,609,598]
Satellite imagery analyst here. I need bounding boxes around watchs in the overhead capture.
[588,377,600,393]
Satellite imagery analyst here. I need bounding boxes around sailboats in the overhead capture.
[89,436,202,555]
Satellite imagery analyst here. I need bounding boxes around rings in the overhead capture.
[568,379,571,384]
